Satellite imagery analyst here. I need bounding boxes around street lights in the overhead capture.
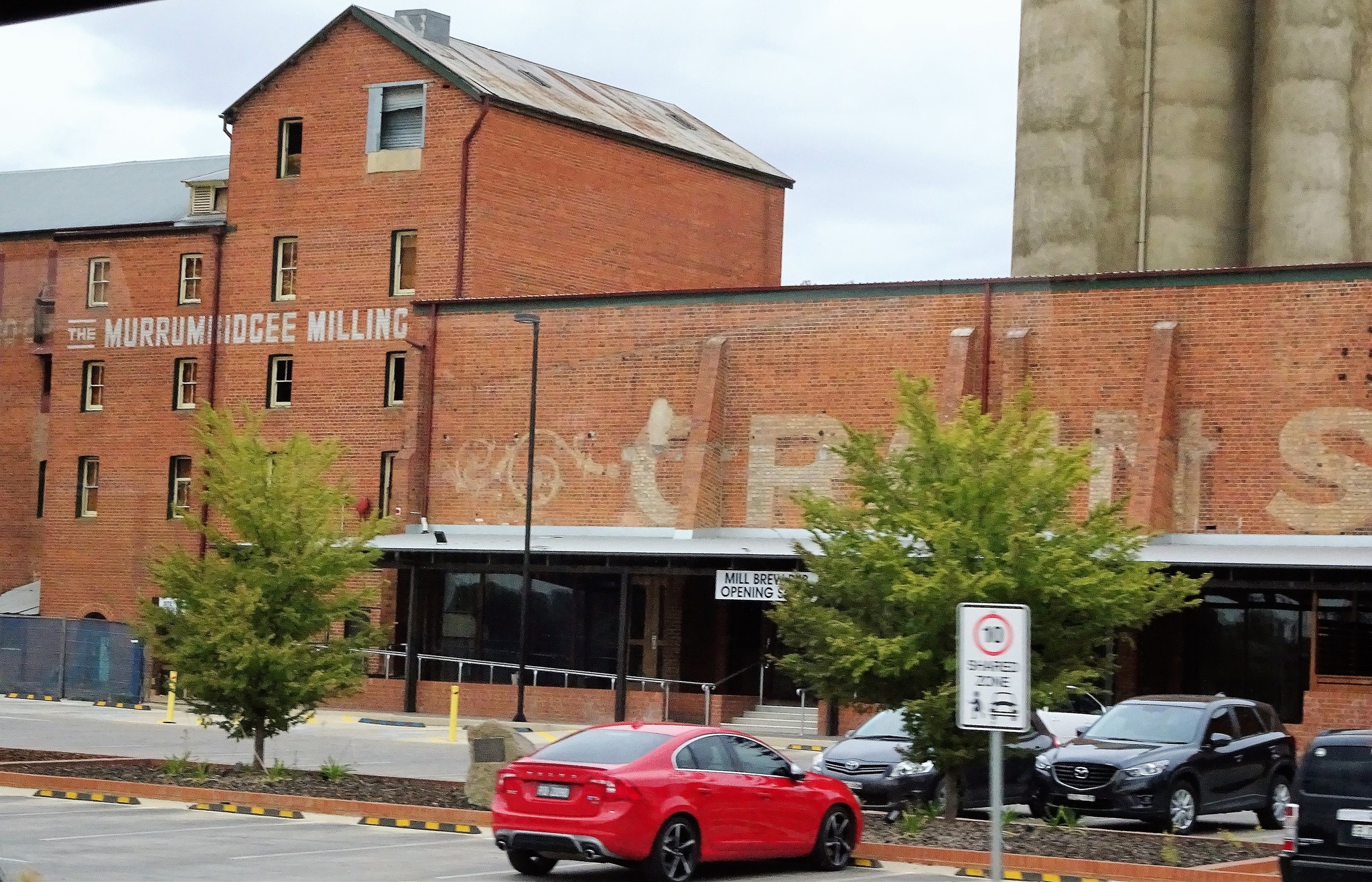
[514,311,545,720]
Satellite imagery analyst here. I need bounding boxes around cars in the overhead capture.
[1025,690,1299,830]
[491,719,866,882]
[807,700,1061,819]
[1278,728,1372,882]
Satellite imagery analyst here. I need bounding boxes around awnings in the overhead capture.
[210,525,1371,574]
